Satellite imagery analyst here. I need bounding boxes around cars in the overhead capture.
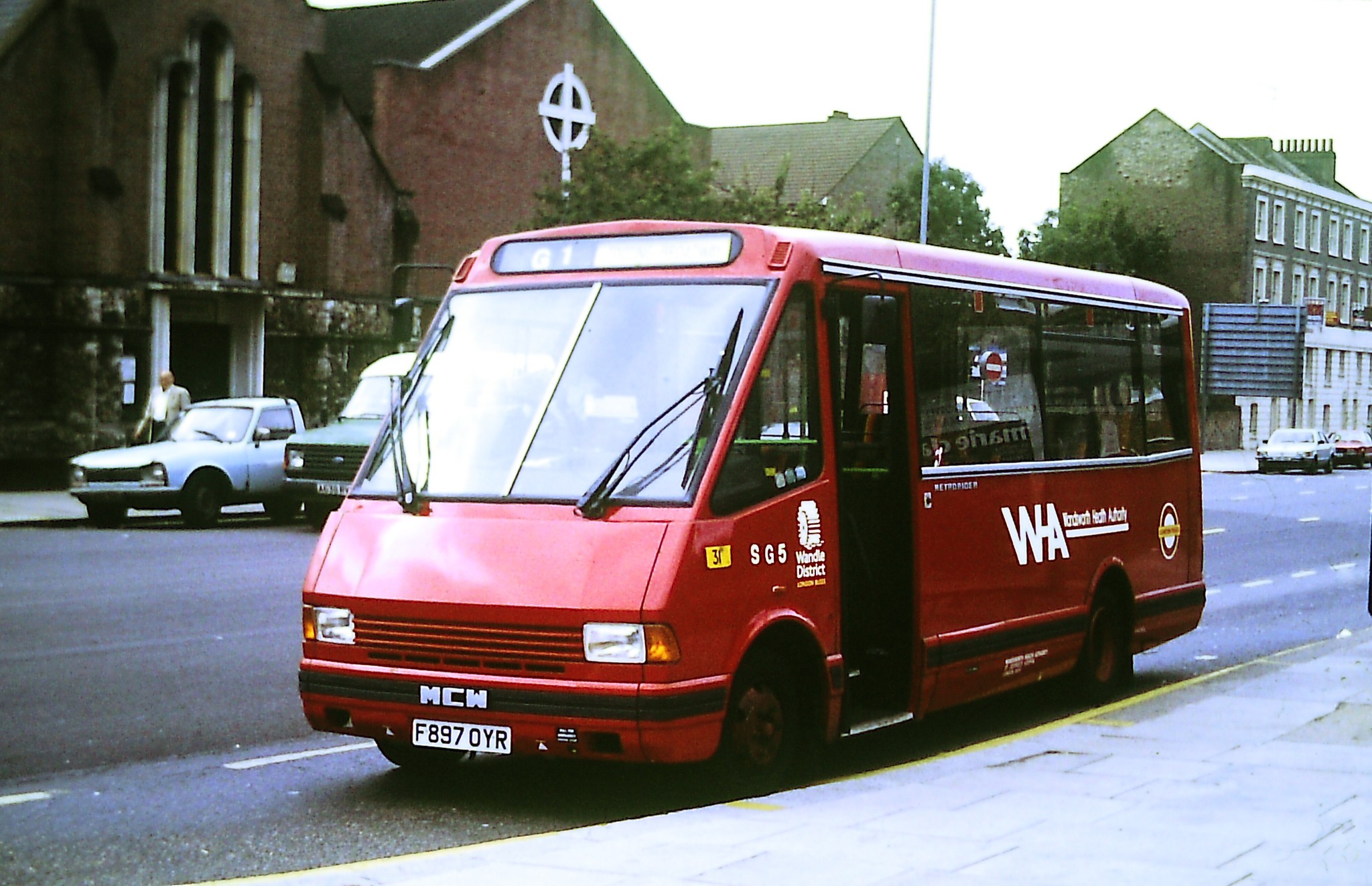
[1254,427,1337,475]
[1328,430,1372,467]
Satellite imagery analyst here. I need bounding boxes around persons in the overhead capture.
[135,370,190,443]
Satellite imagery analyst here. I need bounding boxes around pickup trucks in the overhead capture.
[65,397,311,531]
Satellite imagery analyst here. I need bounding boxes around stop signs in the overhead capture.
[986,352,1004,381]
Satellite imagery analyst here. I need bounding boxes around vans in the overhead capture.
[283,351,428,516]
[293,219,1206,804]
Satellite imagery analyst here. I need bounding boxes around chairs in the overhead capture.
[855,403,888,434]
